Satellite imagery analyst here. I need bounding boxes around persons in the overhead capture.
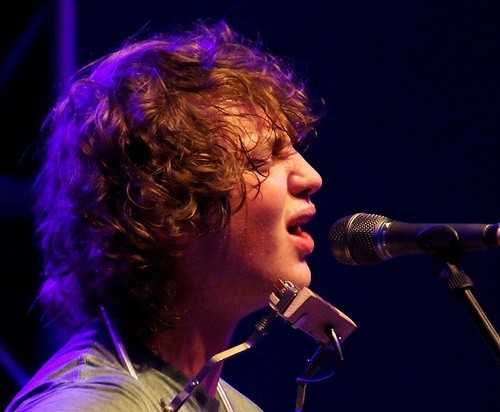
[6,22,322,412]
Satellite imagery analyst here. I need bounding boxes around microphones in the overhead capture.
[327,212,500,266]
